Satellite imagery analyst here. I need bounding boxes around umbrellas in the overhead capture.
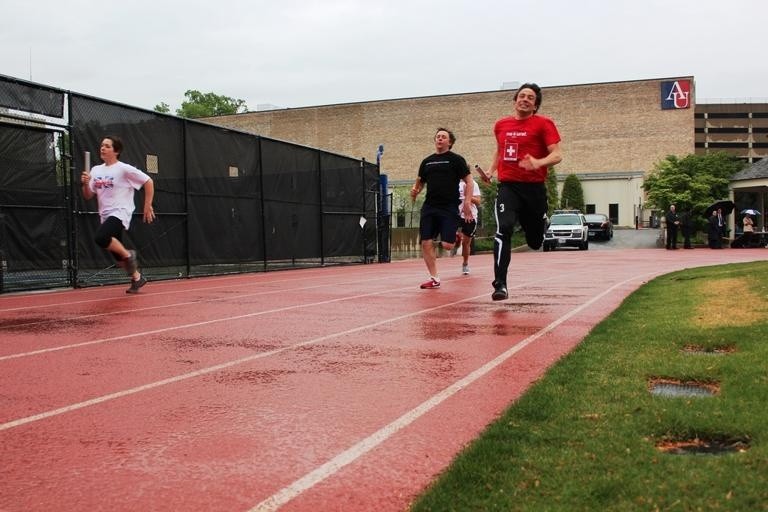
[740,209,762,215]
[704,200,736,220]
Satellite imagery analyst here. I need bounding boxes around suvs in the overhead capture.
[544,209,590,250]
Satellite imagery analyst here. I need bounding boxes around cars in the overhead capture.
[582,213,613,241]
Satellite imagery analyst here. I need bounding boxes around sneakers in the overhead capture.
[449,247,457,257]
[420,277,440,289]
[492,279,508,300]
[123,249,137,275]
[126,273,148,294]
[543,212,551,232]
[461,264,470,275]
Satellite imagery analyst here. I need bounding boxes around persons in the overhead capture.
[410,127,474,288]
[81,136,156,294]
[682,217,692,248]
[665,205,681,250]
[742,214,754,248]
[450,163,481,274]
[482,82,562,300]
[708,207,727,249]
